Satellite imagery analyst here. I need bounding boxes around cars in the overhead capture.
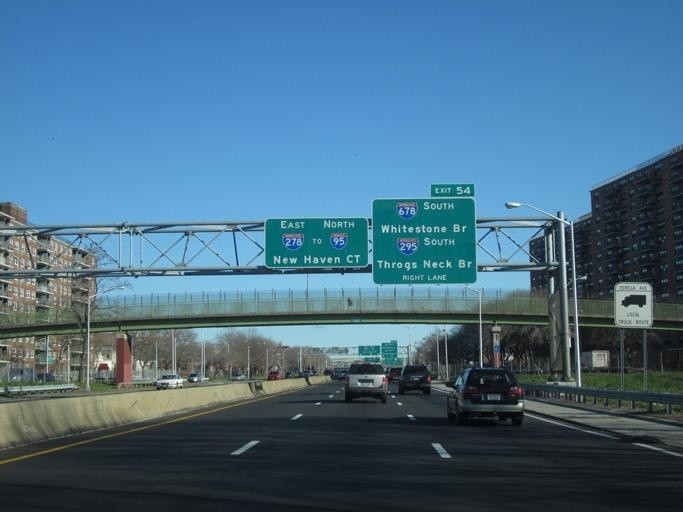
[187,371,282,383]
[398,362,432,395]
[156,373,184,389]
[445,365,525,426]
[344,360,389,404]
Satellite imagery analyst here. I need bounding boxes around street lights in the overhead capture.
[85,284,126,392]
[504,201,583,402]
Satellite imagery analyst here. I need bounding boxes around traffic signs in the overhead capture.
[263,216,369,268]
[358,340,404,365]
[372,196,478,285]
